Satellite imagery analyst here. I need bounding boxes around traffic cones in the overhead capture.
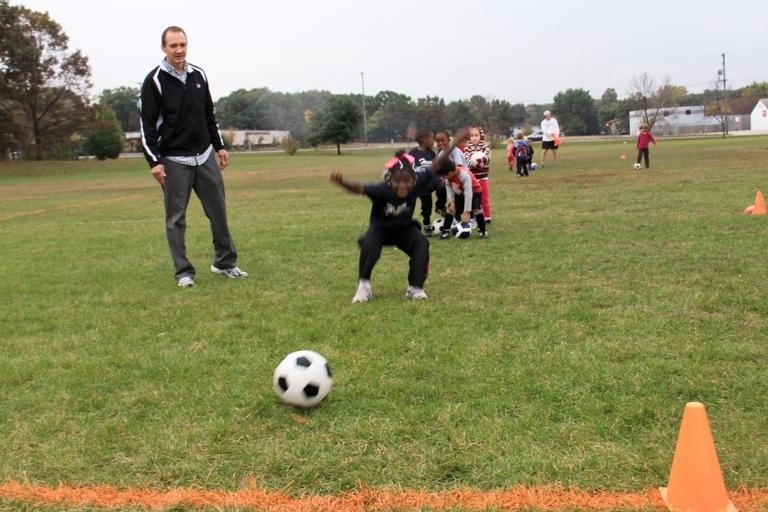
[750,188,768,218]
[655,396,744,512]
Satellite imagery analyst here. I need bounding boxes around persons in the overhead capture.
[540,111,559,168]
[636,124,656,168]
[328,124,493,301]
[507,133,534,176]
[139,25,248,289]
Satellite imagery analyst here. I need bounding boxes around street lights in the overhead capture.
[360,70,369,148]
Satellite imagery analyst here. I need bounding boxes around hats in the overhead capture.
[544,111,551,115]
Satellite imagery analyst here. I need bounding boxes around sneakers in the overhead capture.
[439,230,450,239]
[177,275,195,288]
[478,229,488,238]
[484,216,492,224]
[351,284,373,305]
[210,264,249,278]
[405,284,428,300]
[422,226,432,237]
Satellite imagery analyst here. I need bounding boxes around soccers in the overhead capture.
[531,163,537,169]
[274,350,333,407]
[451,222,472,239]
[633,163,641,170]
[432,219,445,236]
[471,152,485,168]
